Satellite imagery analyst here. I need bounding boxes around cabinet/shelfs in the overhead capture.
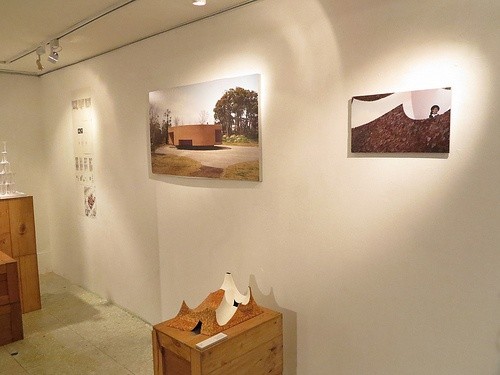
[0,249,25,347]
[0,189,43,315]
[151,303,284,375]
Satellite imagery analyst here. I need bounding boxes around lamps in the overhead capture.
[46,38,63,65]
